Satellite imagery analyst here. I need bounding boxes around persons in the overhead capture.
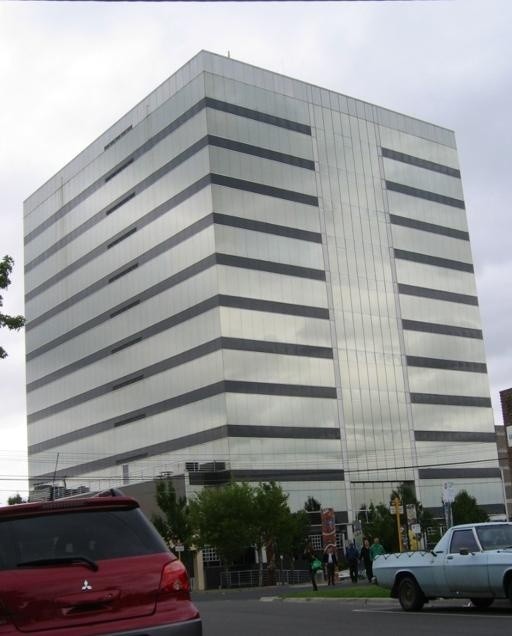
[359,540,377,582]
[344,541,359,583]
[323,544,339,586]
[370,537,388,561]
[306,552,320,593]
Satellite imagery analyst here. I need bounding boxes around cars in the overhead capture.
[1,488,203,636]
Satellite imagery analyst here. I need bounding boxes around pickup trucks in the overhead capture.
[371,522,512,612]
